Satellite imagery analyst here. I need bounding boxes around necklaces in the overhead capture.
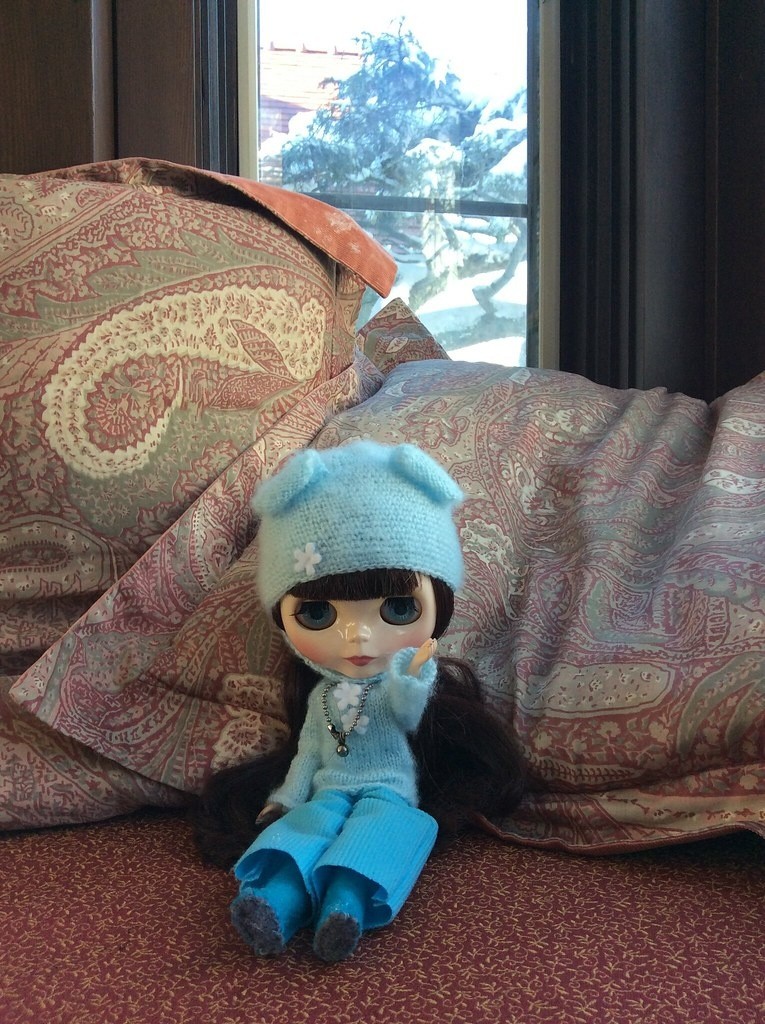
[322,681,372,757]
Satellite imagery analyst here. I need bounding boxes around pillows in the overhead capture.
[5,298,765,852]
[0,156,396,839]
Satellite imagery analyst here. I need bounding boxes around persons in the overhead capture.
[191,440,535,961]
